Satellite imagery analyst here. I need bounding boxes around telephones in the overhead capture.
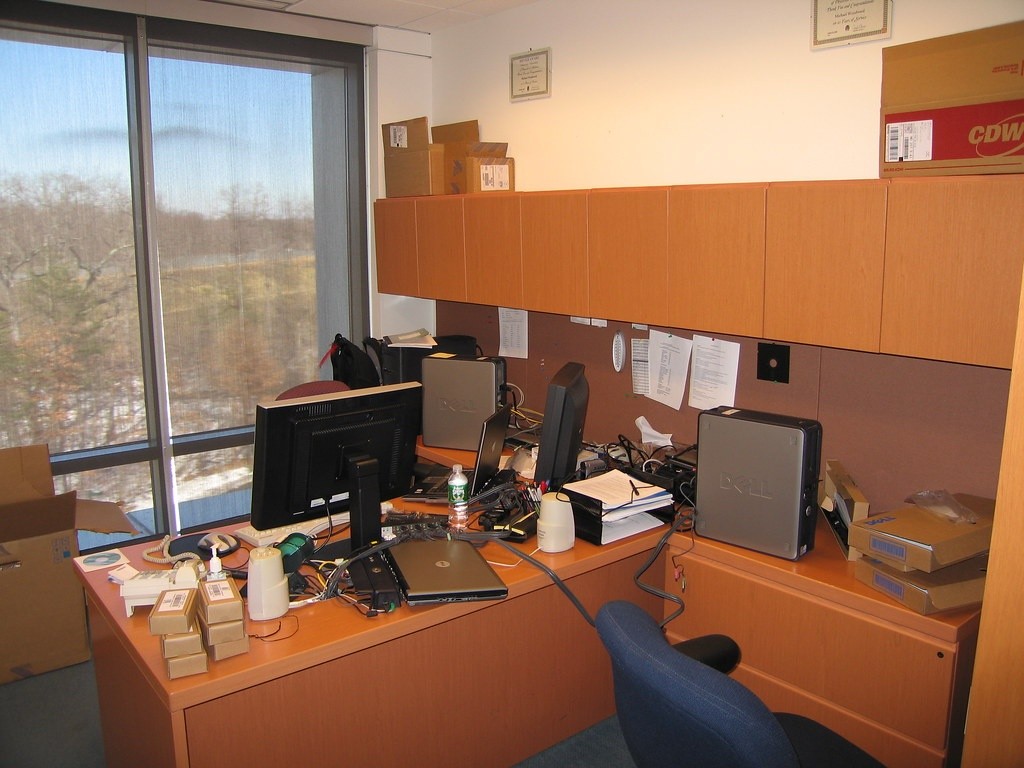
[120,560,207,617]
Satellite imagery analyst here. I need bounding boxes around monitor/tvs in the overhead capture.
[533,362,589,489]
[250,381,422,616]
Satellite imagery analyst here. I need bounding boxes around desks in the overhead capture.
[65,478,694,768]
[416,434,516,472]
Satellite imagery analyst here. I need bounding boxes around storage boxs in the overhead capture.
[0,442,138,686]
[855,547,916,573]
[202,633,249,662]
[382,116,479,197]
[197,609,245,646]
[166,649,208,679]
[878,19,1024,178]
[823,458,870,565]
[847,491,997,573]
[852,554,989,615]
[161,616,203,659]
[197,577,244,625]
[444,140,516,196]
[148,588,198,635]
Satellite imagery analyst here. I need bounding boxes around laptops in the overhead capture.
[403,402,513,503]
[382,539,509,607]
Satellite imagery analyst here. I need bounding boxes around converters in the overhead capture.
[478,507,510,529]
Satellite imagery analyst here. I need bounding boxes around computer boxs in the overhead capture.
[382,335,477,386]
[693,406,823,562]
[422,354,506,451]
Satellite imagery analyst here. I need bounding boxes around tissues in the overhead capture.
[631,415,688,468]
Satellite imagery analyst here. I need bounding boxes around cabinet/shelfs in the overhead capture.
[661,510,988,768]
[373,176,1024,368]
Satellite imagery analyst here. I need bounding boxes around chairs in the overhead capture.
[595,598,890,768]
[275,380,351,401]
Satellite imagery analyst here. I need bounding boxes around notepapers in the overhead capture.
[109,562,140,584]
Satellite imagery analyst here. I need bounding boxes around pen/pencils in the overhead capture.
[518,481,538,502]
[629,480,639,496]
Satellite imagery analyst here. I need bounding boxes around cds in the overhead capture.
[83,553,121,566]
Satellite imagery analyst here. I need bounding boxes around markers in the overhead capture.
[536,487,543,501]
[540,480,547,494]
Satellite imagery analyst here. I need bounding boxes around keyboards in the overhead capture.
[234,501,393,548]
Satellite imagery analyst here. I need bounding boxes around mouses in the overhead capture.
[198,532,238,554]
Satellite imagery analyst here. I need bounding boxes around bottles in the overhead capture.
[447,463,469,532]
